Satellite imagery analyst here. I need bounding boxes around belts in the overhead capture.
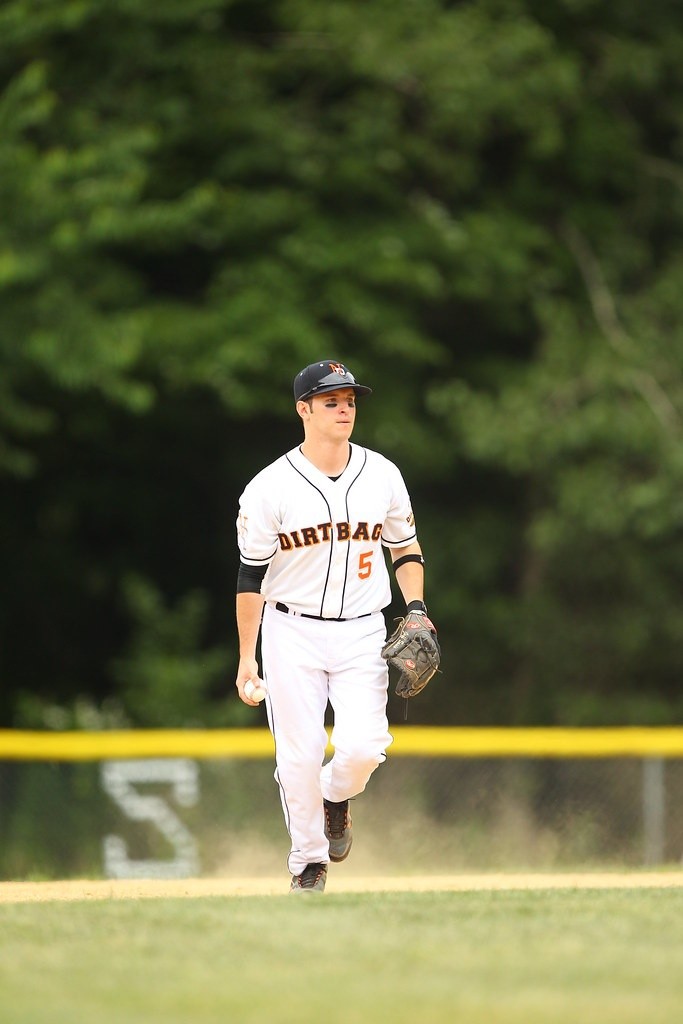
[276,602,371,621]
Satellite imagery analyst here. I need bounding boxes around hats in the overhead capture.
[294,360,372,404]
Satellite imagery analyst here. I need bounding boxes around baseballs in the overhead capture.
[244,677,268,703]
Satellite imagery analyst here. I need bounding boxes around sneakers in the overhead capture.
[289,861,328,895]
[323,797,353,862]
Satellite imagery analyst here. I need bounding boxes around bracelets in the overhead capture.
[408,601,427,613]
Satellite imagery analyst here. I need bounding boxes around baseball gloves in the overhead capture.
[379,609,442,701]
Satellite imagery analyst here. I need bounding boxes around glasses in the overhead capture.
[297,372,356,400]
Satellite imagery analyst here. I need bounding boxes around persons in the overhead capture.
[236,361,441,891]
